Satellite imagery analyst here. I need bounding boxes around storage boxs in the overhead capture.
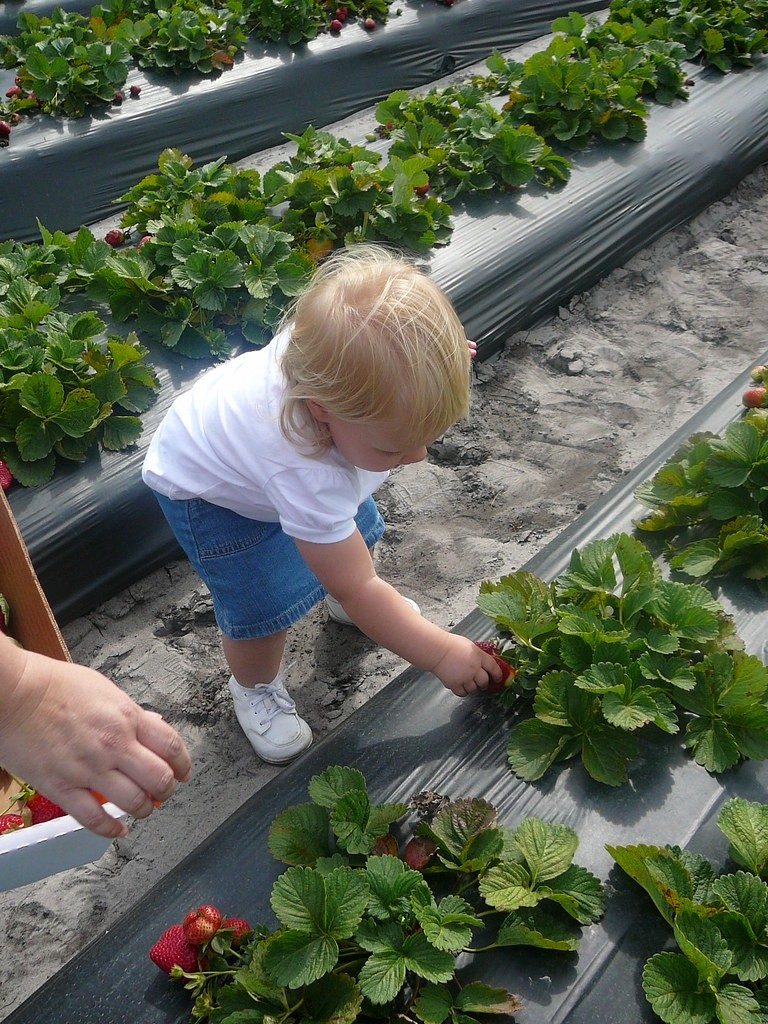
[0,481,126,891]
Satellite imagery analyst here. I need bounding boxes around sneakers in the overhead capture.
[325,591,421,627]
[227,674,312,765]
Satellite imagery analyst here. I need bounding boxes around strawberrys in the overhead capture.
[751,365,767,381]
[488,656,510,690]
[474,641,492,654]
[184,906,220,944]
[104,230,125,246]
[387,121,394,128]
[743,388,766,407]
[5,770,65,819]
[137,235,152,249]
[0,460,12,490]
[150,925,196,975]
[365,134,376,141]
[0,814,23,835]
[220,917,248,942]
[0,6,375,135]
[413,182,429,194]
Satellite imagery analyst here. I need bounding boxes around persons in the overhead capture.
[0,631,190,838]
[136,245,502,764]
